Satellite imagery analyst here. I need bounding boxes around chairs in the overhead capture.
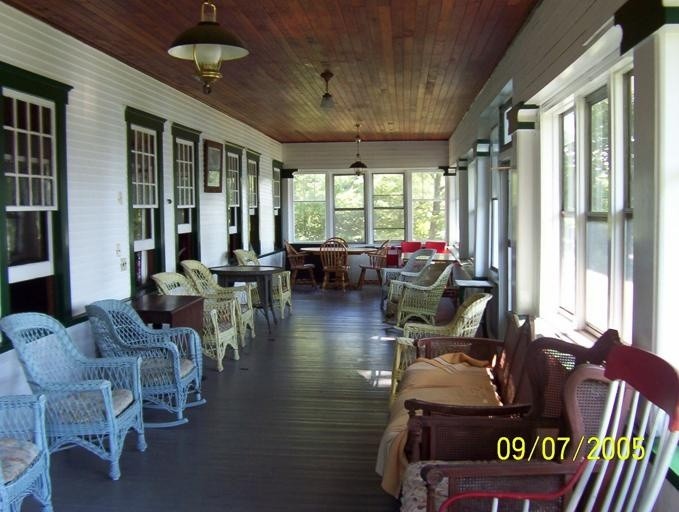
[379,248,437,317]
[398,329,623,512]
[386,262,454,326]
[438,343,679,512]
[0,394,55,512]
[387,292,494,417]
[326,236,352,288]
[319,240,352,293]
[357,239,390,289]
[283,239,318,291]
[181,259,256,350]
[233,249,293,319]
[401,241,421,254]
[150,271,240,373]
[1,311,148,480]
[85,298,207,430]
[426,241,446,252]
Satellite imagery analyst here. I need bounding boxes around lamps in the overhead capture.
[320,70,336,109]
[167,0,249,96]
[348,124,368,176]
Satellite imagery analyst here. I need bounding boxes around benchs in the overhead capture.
[375,312,548,500]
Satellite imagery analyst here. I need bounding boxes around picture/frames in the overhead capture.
[203,139,223,193]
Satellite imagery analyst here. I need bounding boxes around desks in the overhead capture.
[299,247,378,290]
[132,293,205,382]
[207,265,283,334]
[455,278,496,338]
[401,252,457,286]
